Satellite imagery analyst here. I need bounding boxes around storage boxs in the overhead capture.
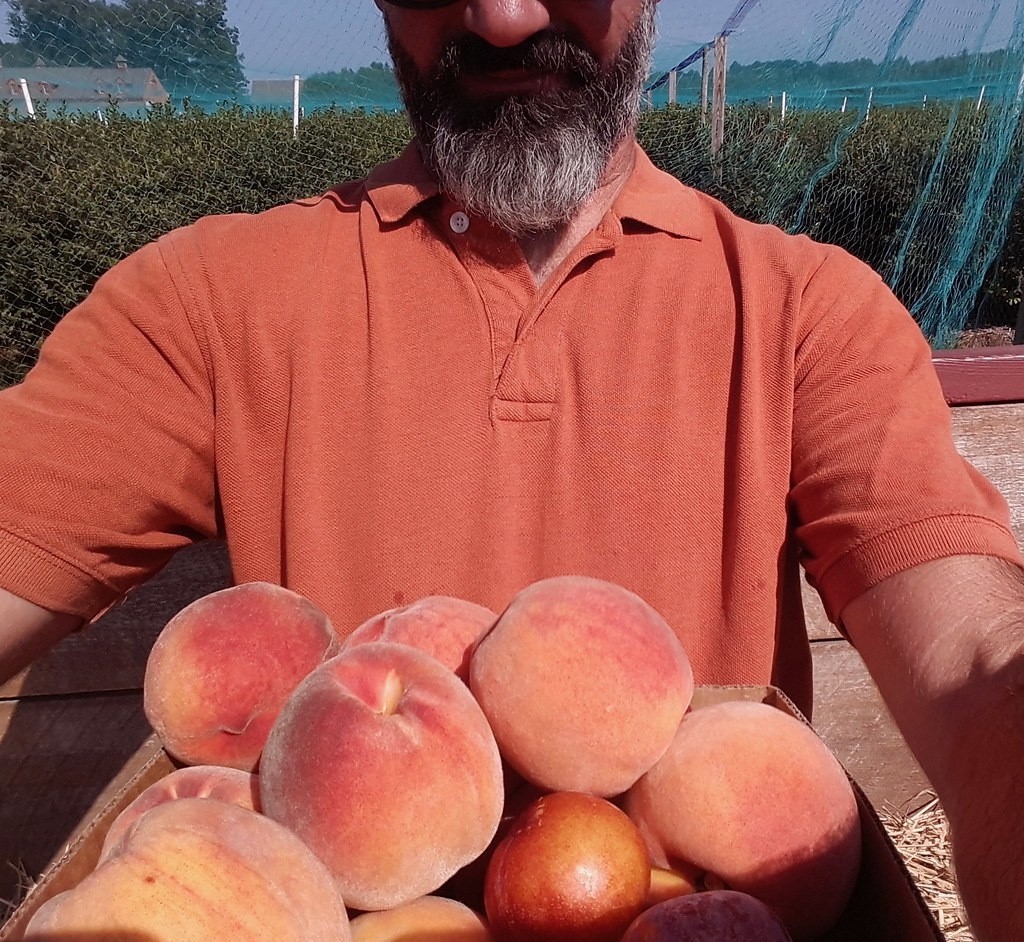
[0,685,947,942]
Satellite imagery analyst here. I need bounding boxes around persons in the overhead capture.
[0,0,1024,941]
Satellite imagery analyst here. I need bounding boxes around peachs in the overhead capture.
[21,575,863,942]
